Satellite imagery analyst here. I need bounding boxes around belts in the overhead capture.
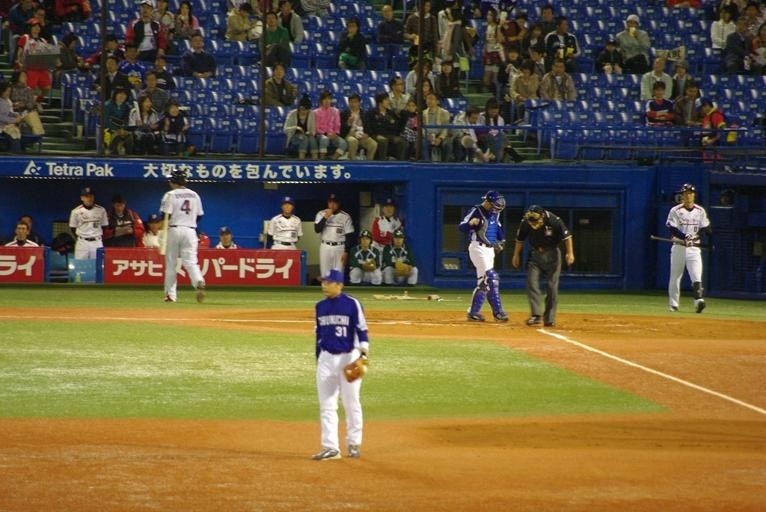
[84,237,95,241]
[322,240,343,246]
[281,241,296,245]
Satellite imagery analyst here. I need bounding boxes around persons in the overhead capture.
[312,269,368,459]
[267,192,418,287]
[665,183,711,314]
[511,205,575,328]
[3,215,46,248]
[70,185,162,261]
[0,0,766,166]
[452,189,509,325]
[157,171,206,305]
[195,226,239,250]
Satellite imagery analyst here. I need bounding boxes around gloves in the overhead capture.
[684,233,697,241]
[684,238,693,247]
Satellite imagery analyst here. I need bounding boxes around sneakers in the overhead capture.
[348,444,359,458]
[669,306,679,312]
[526,315,540,325]
[468,313,485,322]
[694,298,705,313]
[312,448,341,460]
[494,311,508,321]
[544,322,555,326]
[195,280,207,304]
[164,294,172,302]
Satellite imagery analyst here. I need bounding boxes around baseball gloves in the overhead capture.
[362,260,376,271]
[394,262,412,276]
[344,352,369,383]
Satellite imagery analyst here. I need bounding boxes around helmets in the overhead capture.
[523,205,544,231]
[678,183,696,194]
[481,190,505,216]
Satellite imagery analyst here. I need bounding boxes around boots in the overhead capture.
[504,146,528,163]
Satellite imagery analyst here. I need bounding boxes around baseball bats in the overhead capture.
[650,235,715,249]
[159,192,173,256]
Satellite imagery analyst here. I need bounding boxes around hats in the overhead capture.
[695,96,708,112]
[675,59,689,69]
[316,269,345,283]
[627,14,641,25]
